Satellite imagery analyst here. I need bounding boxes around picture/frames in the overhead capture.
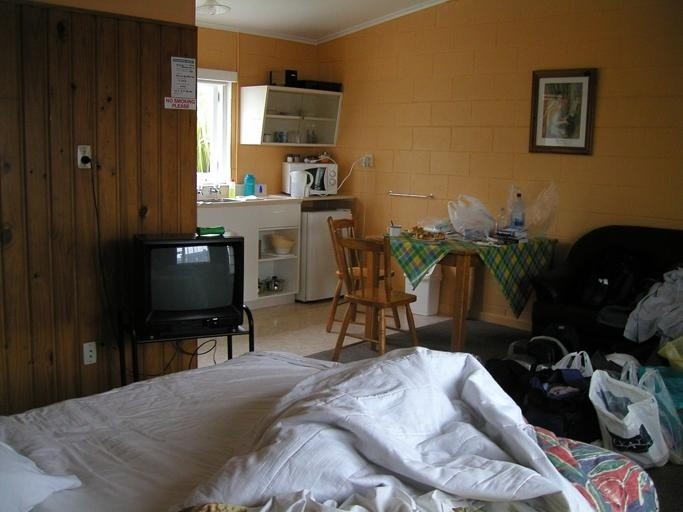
[527,67,598,156]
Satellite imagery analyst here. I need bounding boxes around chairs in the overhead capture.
[326,216,417,361]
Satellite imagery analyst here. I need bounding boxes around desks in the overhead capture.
[365,231,558,352]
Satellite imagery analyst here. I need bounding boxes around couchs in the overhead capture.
[529,224,682,376]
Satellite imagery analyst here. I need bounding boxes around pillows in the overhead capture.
[0,441,81,511]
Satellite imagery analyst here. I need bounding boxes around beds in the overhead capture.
[0,350,659,511]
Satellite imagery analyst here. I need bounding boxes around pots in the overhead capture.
[265,274,285,292]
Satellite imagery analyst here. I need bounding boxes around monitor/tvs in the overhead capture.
[126,235,244,339]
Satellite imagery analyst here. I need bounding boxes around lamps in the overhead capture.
[196,0,231,18]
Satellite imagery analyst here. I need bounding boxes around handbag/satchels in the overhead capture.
[588,369,668,469]
[521,355,600,441]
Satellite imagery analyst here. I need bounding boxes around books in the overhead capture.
[494,227,530,244]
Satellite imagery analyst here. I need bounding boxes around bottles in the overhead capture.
[244,174,256,197]
[262,129,320,143]
[511,192,526,230]
[496,208,507,230]
[287,153,301,163]
[228,181,236,198]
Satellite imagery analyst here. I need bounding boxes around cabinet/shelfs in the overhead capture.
[239,85,343,147]
[118,304,254,386]
[257,203,300,308]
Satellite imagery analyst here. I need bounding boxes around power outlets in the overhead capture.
[76,144,91,168]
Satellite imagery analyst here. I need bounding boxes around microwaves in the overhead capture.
[280,162,339,195]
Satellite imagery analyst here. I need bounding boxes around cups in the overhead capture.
[254,183,267,199]
[386,224,402,237]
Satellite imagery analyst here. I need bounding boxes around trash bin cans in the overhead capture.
[403,263,442,316]
